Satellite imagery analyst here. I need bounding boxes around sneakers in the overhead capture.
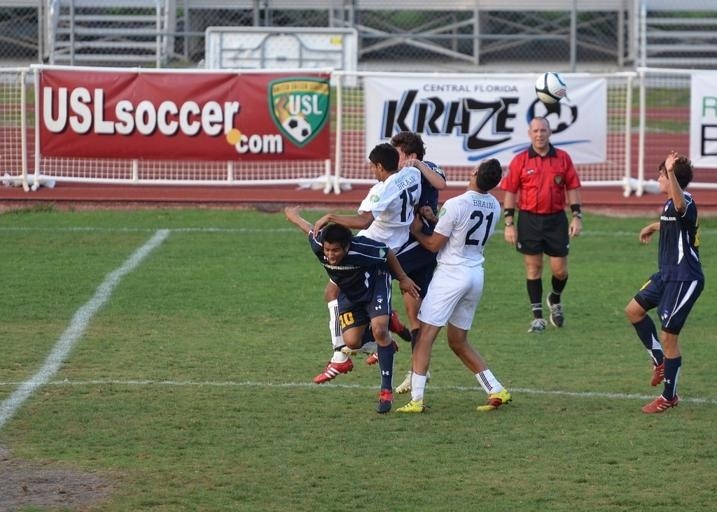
[396,400,426,413]
[377,386,393,413]
[651,363,667,387]
[395,369,430,394]
[546,291,565,329]
[366,341,398,365]
[313,357,354,385]
[476,389,513,412]
[527,318,547,333]
[388,310,412,342]
[642,393,679,413]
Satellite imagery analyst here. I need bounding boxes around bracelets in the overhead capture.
[569,203,581,213]
[504,208,515,217]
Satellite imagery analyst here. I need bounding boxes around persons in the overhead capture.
[394,158,512,413]
[625,150,705,414]
[496,116,584,333]
[284,205,421,413]
[389,131,446,394]
[312,143,422,383]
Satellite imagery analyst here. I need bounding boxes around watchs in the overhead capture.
[572,213,583,218]
[505,221,514,226]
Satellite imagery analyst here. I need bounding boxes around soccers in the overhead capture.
[535,73,567,104]
